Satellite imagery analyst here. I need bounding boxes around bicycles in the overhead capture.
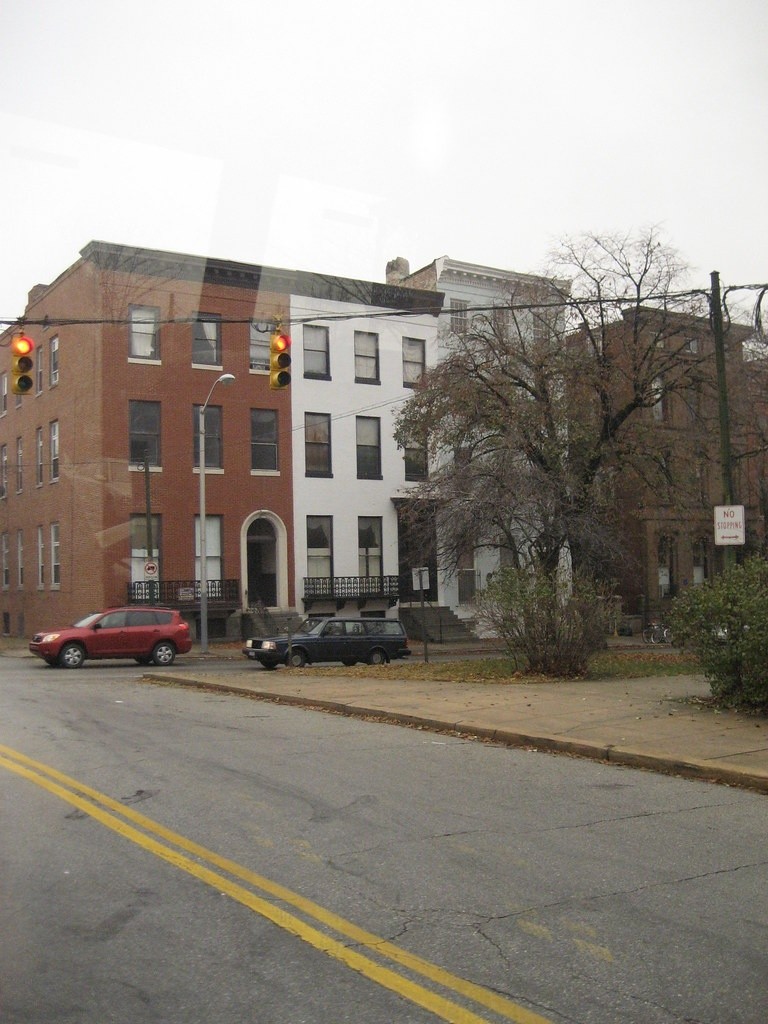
[642,621,675,645]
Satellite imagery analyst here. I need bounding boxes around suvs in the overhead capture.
[29,606,192,668]
[241,615,412,671]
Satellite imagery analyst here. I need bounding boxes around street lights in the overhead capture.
[199,373,237,654]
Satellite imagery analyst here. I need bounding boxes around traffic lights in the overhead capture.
[268,333,291,389]
[11,334,35,397]
